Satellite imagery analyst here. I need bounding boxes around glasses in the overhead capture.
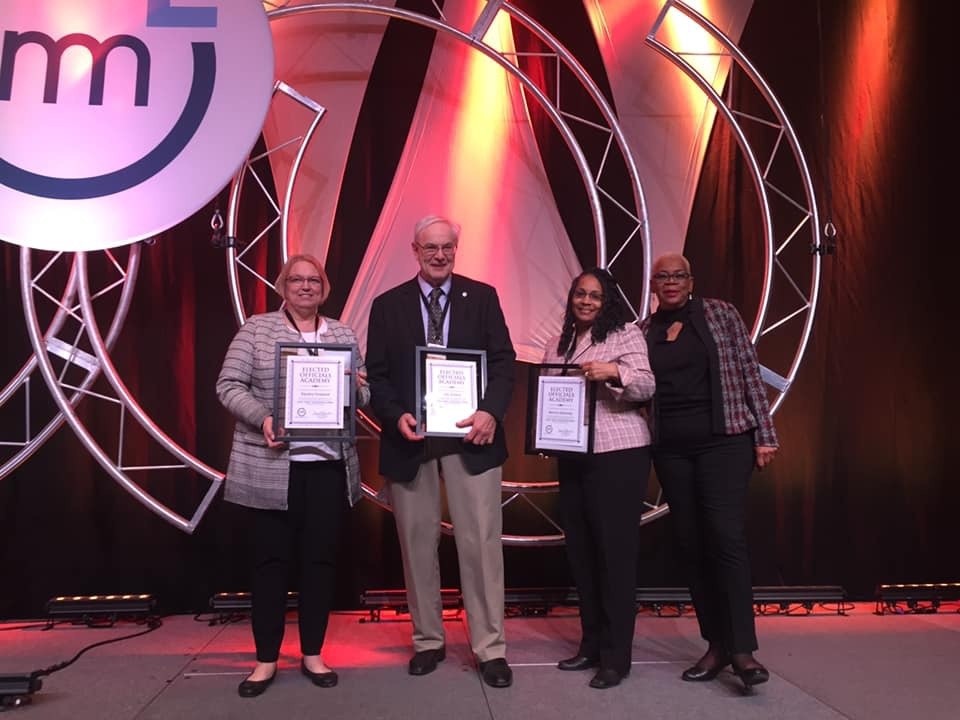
[286,276,322,287]
[417,241,456,254]
[655,273,690,282]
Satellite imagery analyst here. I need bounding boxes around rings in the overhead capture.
[482,437,488,441]
[585,372,589,376]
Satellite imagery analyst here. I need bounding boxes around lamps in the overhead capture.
[503,588,580,618]
[208,590,299,625]
[359,589,464,624]
[873,582,960,616]
[633,587,694,617]
[751,585,855,617]
[43,593,161,628]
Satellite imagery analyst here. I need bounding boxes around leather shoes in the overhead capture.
[409,646,444,675]
[590,667,628,688]
[480,657,512,686]
[558,655,596,670]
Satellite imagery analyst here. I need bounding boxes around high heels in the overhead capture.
[730,656,770,691]
[682,653,730,681]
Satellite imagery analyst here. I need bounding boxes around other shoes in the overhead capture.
[237,668,277,696]
[301,657,337,687]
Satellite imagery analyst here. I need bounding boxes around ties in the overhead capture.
[427,288,444,345]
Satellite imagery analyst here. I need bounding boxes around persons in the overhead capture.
[365,216,518,687]
[216,254,370,698]
[539,267,655,688]
[638,253,781,694]
